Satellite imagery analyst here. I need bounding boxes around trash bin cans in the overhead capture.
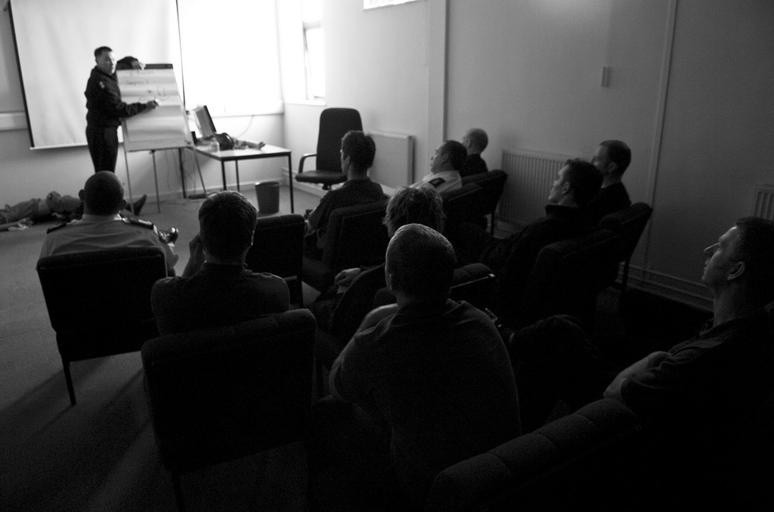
[255,181,280,214]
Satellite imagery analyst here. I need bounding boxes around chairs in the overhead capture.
[294,108,363,194]
[189,169,655,358]
[37,244,170,407]
[139,306,314,507]
[427,396,645,511]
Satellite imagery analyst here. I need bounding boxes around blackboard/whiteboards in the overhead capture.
[0,0,282,132]
[303,0,435,100]
[115,69,194,152]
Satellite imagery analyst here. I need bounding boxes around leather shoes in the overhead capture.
[125,195,147,216]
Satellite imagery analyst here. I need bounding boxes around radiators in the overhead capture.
[494,144,580,227]
[363,129,415,190]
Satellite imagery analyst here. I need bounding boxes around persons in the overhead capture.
[85,46,157,170]
[150,191,291,339]
[40,170,179,278]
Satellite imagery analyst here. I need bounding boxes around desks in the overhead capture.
[177,141,295,213]
